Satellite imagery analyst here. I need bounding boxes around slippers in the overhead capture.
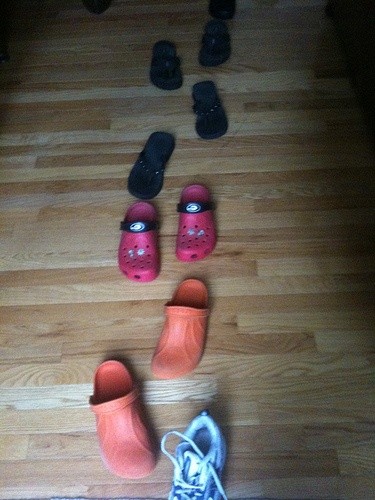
[149,40,182,89]
[149,278,209,378]
[118,201,161,284]
[89,360,156,479]
[127,131,176,200]
[199,20,232,67]
[175,183,217,262]
[192,80,229,140]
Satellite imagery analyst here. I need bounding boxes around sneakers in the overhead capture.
[168,411,225,500]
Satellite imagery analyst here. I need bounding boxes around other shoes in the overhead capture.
[82,0,112,15]
[209,0,236,20]
